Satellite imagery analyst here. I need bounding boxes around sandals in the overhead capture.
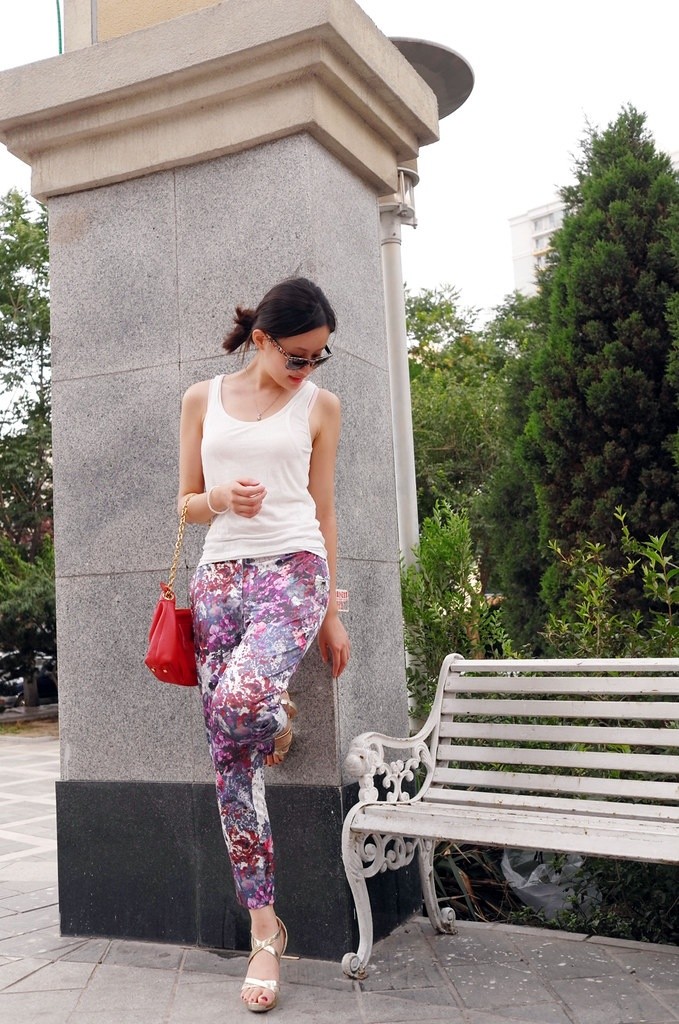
[263,690,297,767]
[242,916,288,1012]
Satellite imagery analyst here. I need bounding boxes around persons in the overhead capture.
[178,274,352,1012]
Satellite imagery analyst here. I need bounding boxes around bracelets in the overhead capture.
[207,487,230,516]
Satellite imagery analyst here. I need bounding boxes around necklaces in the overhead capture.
[244,364,283,421]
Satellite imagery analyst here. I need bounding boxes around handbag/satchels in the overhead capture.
[143,494,198,687]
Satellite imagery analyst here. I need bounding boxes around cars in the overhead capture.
[0,650,59,713]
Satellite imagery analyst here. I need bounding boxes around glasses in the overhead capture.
[263,329,333,370]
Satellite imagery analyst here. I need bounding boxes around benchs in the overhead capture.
[339,653,679,981]
